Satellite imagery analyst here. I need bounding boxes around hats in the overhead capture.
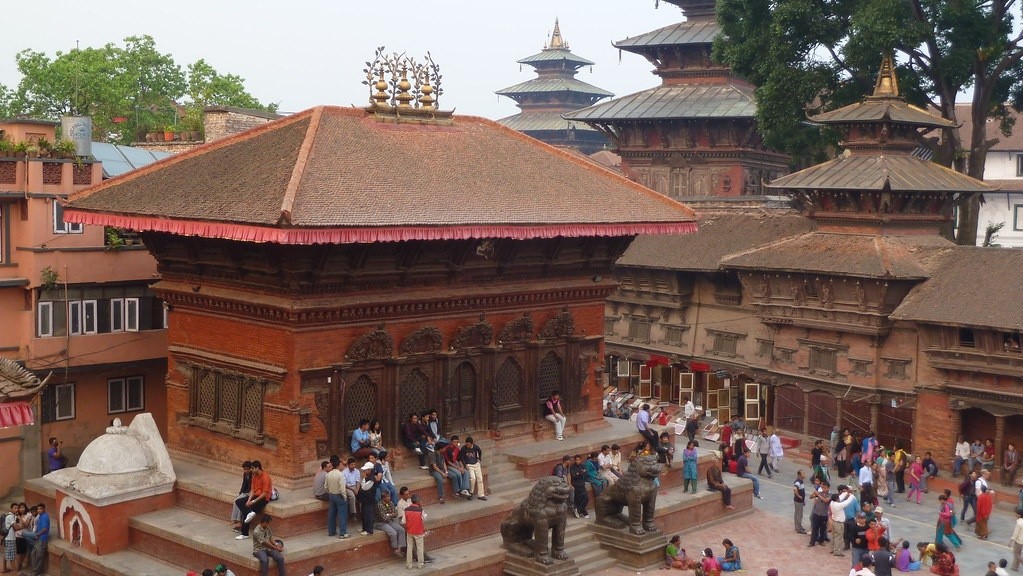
[187,570,199,576]
[361,462,375,470]
[214,564,226,572]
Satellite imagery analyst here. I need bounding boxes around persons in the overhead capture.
[544,389,566,441]
[202,564,236,576]
[48,438,65,471]
[307,565,324,576]
[717,539,741,572]
[252,515,286,576]
[554,395,783,519]
[767,568,779,576]
[404,410,488,502]
[231,460,272,540]
[666,535,698,569]
[313,451,434,569]
[695,548,722,576]
[1,502,50,576]
[368,419,386,454]
[793,426,1023,576]
[351,418,380,458]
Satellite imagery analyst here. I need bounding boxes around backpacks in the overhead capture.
[0,514,15,538]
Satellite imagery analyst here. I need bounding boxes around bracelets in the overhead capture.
[445,471,448,473]
[276,543,278,546]
[556,417,558,419]
[252,500,255,504]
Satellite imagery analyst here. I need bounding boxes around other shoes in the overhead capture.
[844,546,850,550]
[416,558,430,563]
[339,533,351,539]
[28,572,43,576]
[885,499,896,508]
[754,492,765,500]
[232,527,242,533]
[460,489,472,497]
[451,492,461,499]
[571,509,590,519]
[875,507,884,513]
[468,492,487,501]
[235,535,249,540]
[426,444,434,452]
[1002,481,1012,487]
[924,488,928,493]
[555,434,563,441]
[244,511,256,524]
[816,536,830,542]
[795,527,807,534]
[438,496,444,504]
[413,447,423,454]
[394,552,404,558]
[357,528,374,536]
[806,542,826,547]
[766,568,779,576]
[683,489,696,494]
[420,465,428,470]
[829,552,845,557]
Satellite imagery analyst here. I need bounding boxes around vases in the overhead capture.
[181,130,198,141]
[125,239,133,246]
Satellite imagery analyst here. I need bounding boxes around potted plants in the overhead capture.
[0,133,77,159]
[145,126,176,140]
